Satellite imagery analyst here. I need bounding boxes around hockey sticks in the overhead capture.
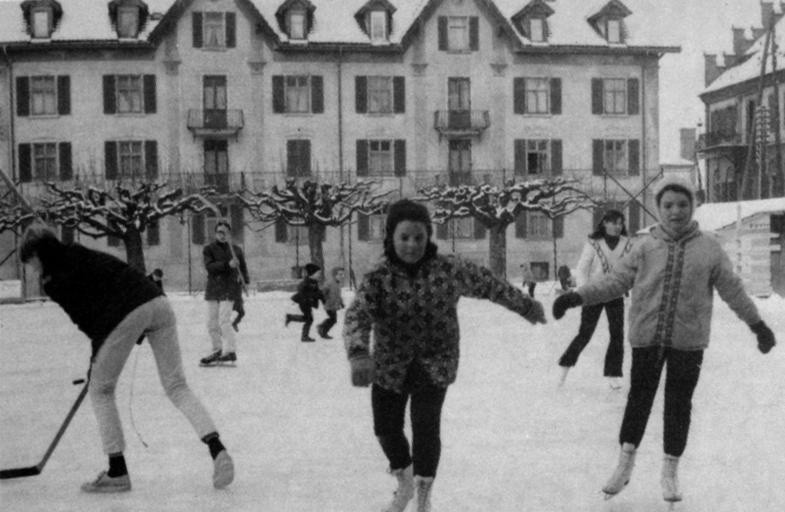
[1,382,89,478]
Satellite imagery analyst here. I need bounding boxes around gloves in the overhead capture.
[554,291,582,319]
[523,297,548,325]
[750,320,774,353]
[349,354,377,386]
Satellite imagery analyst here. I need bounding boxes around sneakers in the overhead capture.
[232,322,238,331]
[201,352,237,363]
[286,313,291,325]
[557,366,570,378]
[81,469,131,492]
[301,324,333,342]
[213,449,235,488]
[609,377,625,390]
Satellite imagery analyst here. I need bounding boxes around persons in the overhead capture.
[517,262,537,297]
[317,266,345,340]
[16,228,235,492]
[285,260,324,342]
[342,197,546,512]
[555,265,577,293]
[558,209,634,390]
[552,174,775,503]
[144,268,164,292]
[201,220,250,366]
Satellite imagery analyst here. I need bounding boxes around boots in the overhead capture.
[412,474,435,512]
[660,453,683,501]
[600,441,636,494]
[384,458,414,512]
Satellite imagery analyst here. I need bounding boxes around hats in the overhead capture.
[304,263,321,275]
[153,269,163,277]
